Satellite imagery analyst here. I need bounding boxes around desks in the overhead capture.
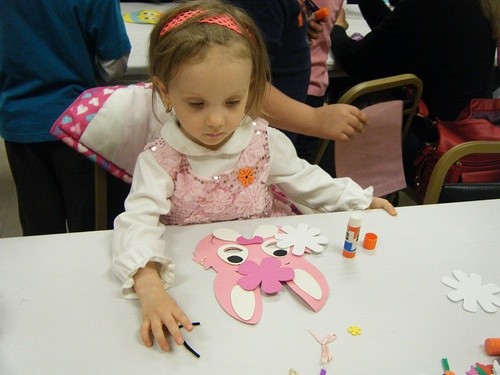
[117,3,180,85]
[0,199,500,375]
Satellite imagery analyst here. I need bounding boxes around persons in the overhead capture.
[224,0,500,178]
[108,0,398,352]
[0,0,132,237]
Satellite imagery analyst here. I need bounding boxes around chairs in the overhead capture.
[94,86,155,231]
[311,74,500,200]
